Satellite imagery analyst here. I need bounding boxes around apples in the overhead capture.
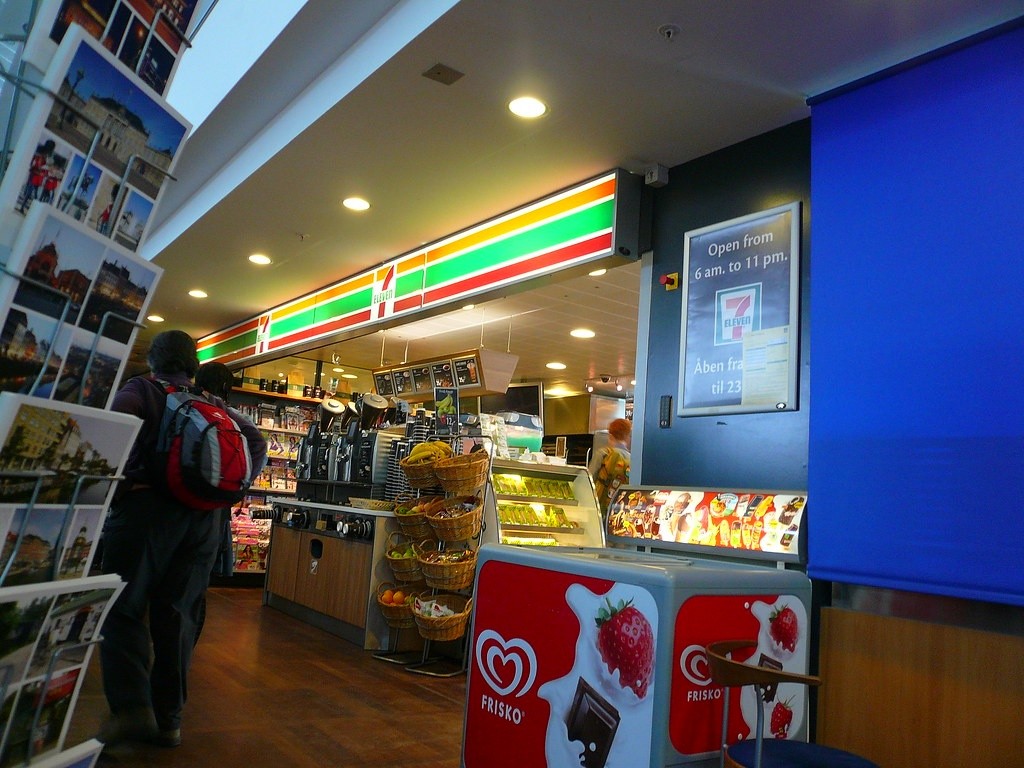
[399,501,431,514]
[391,548,416,558]
[437,406,455,417]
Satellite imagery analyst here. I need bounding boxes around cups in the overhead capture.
[288,513,307,525]
[385,409,462,504]
[250,508,282,520]
[337,520,372,538]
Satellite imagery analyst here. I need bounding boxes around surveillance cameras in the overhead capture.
[601,373,611,382]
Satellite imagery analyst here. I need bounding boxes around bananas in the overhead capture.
[436,394,453,408]
[407,440,454,464]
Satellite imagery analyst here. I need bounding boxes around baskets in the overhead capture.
[425,489,485,541]
[384,532,437,582]
[433,447,490,492]
[410,592,474,641]
[394,493,444,544]
[411,543,479,591]
[377,582,432,629]
[399,448,448,488]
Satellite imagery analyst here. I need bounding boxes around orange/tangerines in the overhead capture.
[381,590,409,605]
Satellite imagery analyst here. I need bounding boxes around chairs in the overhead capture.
[705,639,874,768]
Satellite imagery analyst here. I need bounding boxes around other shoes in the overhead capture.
[97,705,158,748]
[155,726,181,746]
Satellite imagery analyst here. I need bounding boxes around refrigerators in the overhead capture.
[458,485,812,768]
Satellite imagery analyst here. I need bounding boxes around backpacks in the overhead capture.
[134,373,252,510]
[595,445,631,521]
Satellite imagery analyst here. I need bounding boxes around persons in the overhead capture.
[193,362,234,645]
[117,211,132,235]
[269,434,282,455]
[60,543,86,576]
[587,418,637,559]
[609,490,626,523]
[19,153,58,215]
[91,329,267,746]
[96,197,122,237]
[288,436,298,452]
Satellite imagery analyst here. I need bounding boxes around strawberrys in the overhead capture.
[769,603,799,653]
[770,696,797,734]
[595,596,655,699]
